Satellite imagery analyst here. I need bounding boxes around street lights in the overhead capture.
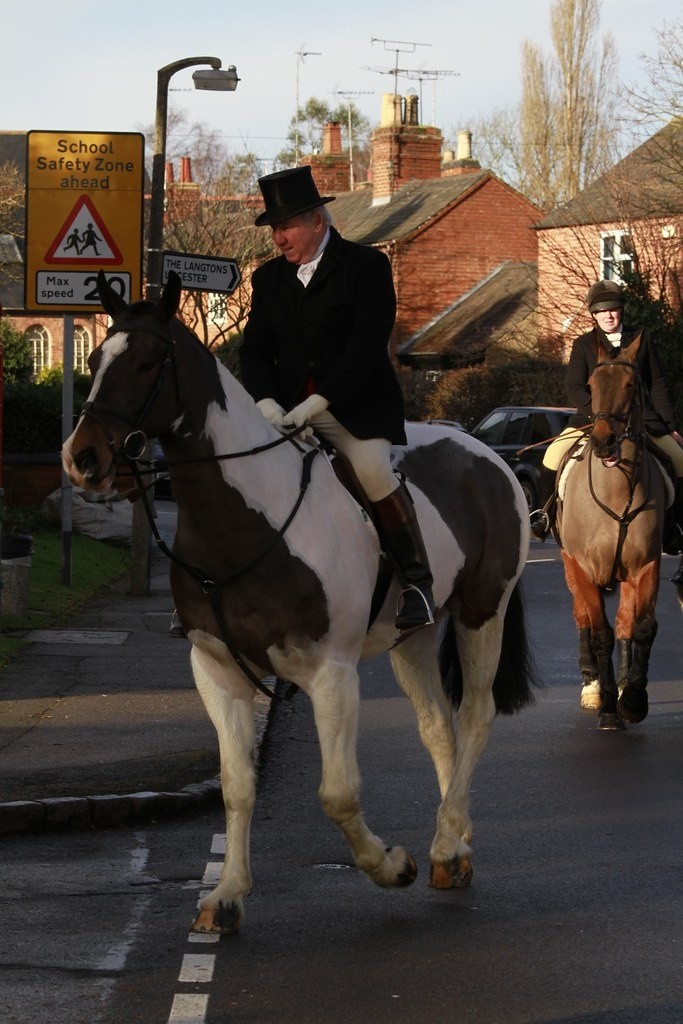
[141,56,242,302]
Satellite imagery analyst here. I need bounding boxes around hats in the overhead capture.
[255,165,335,228]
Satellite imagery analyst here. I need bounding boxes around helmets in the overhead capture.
[587,279,623,314]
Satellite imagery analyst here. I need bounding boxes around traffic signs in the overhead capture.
[161,247,243,294]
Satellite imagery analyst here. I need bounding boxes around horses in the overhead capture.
[62,266,542,939]
[554,326,669,733]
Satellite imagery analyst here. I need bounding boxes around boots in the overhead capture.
[362,475,436,629]
[529,462,558,542]
[169,612,184,637]
[662,477,683,555]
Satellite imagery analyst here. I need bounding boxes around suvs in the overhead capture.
[467,406,580,526]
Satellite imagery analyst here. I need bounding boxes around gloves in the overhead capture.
[283,394,329,440]
[256,398,285,429]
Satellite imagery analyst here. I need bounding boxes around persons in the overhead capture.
[232,166,437,629]
[532,279,683,584]
